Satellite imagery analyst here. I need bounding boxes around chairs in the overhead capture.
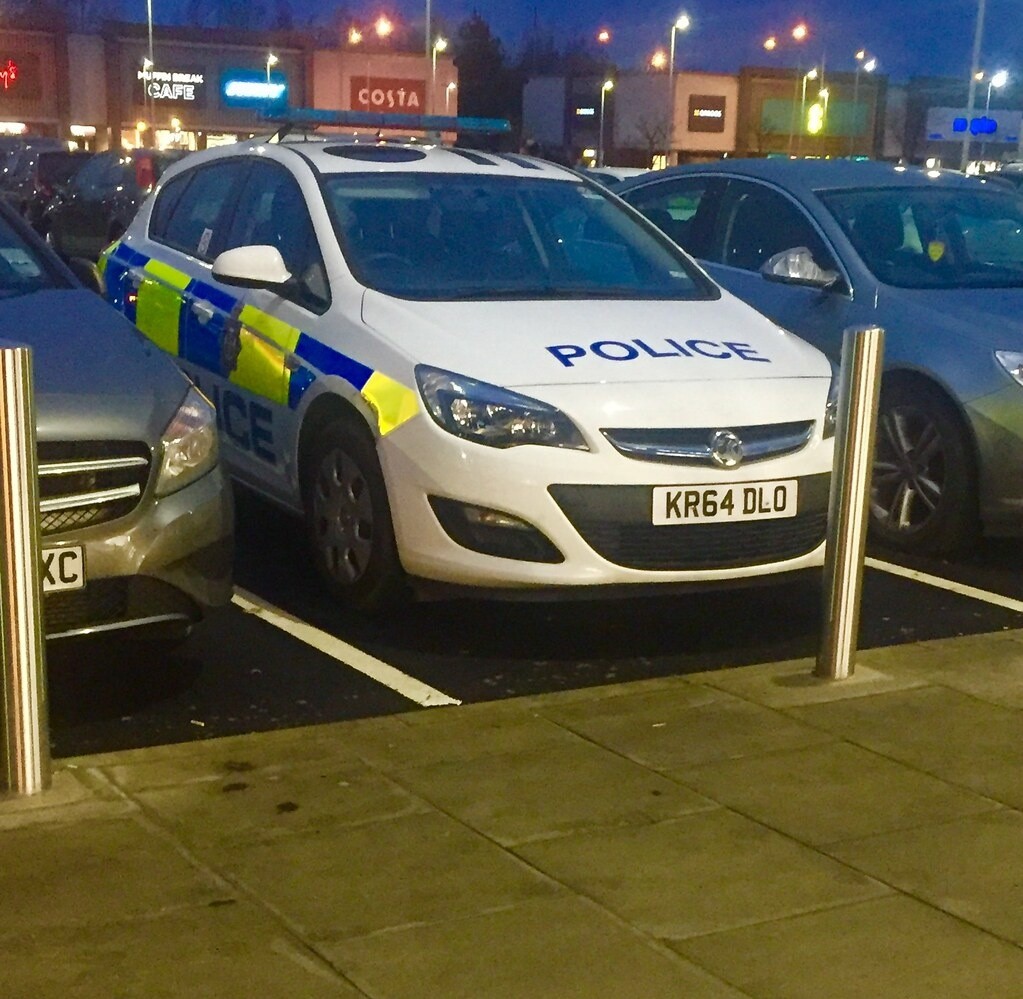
[850,202,943,290]
[747,202,839,276]
[347,198,418,297]
[248,181,322,282]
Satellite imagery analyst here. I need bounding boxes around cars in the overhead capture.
[0,202,236,662]
[93,109,844,637]
[41,148,199,262]
[585,166,651,186]
[940,161,1023,194]
[0,135,96,217]
[605,158,1023,550]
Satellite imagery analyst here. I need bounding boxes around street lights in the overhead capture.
[266,53,278,101]
[598,80,614,168]
[431,37,448,116]
[444,81,457,114]
[787,23,808,158]
[663,14,690,155]
[349,13,396,113]
[978,70,1009,159]
[798,68,817,156]
[849,48,878,159]
[142,57,158,128]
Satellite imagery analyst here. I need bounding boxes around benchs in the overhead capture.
[639,208,707,258]
